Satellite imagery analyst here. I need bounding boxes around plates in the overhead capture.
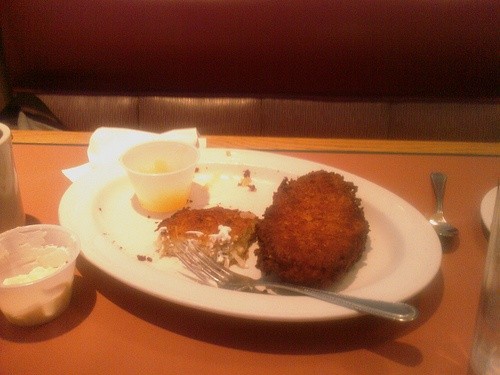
[480,186,497,231]
[59,147,442,323]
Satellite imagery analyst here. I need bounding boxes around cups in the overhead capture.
[0,223,81,326]
[0,123,26,232]
[120,140,199,213]
[467,182,500,375]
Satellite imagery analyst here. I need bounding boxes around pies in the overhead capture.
[156,207,261,267]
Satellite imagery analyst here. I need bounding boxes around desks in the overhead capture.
[0,130,494,375]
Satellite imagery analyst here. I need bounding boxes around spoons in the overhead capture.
[426,172,460,236]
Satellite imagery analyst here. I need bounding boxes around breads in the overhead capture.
[254,170,369,288]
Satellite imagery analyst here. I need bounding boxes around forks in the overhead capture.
[172,239,417,322]
